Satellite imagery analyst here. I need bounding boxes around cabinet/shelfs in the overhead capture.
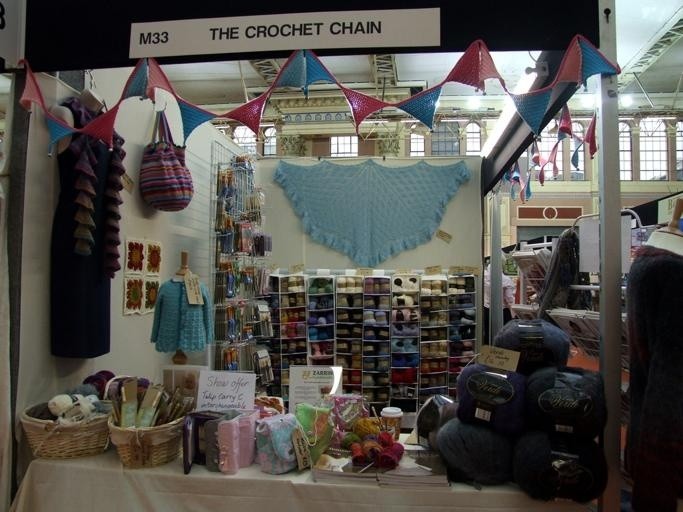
[447,272,480,404]
[483,208,656,493]
[391,272,421,430]
[305,274,334,367]
[418,273,447,414]
[335,274,364,398]
[267,273,309,414]
[363,275,392,411]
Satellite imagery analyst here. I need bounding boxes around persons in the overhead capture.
[47,87,113,360]
[182,372,197,410]
[620,226,682,512]
[482,261,514,345]
[149,268,214,353]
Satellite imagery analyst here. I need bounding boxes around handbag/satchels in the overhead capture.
[253,414,311,471]
[139,111,194,212]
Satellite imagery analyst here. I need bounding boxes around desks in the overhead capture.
[12,432,597,512]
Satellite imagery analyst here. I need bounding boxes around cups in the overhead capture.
[379,405,403,441]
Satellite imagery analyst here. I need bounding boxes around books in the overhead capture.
[309,442,453,492]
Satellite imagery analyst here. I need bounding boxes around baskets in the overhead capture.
[18,401,192,469]
